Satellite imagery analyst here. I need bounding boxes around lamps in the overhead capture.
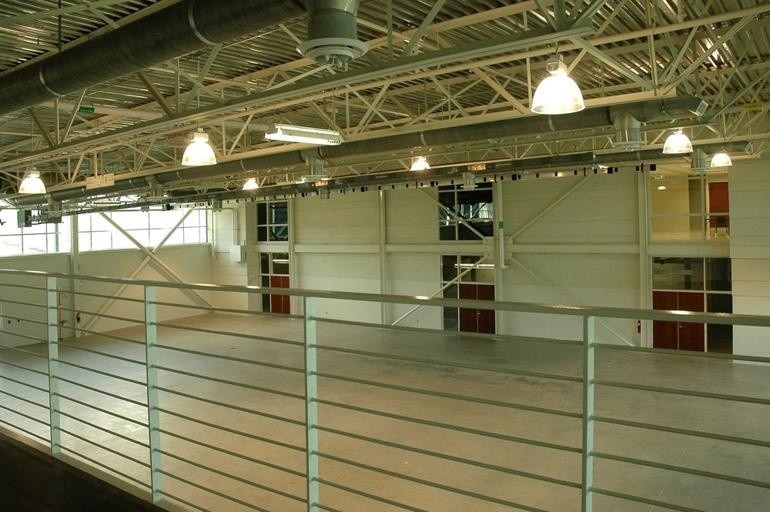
[243,176,259,191]
[183,57,217,166]
[663,128,693,155]
[411,152,431,172]
[711,143,732,167]
[529,41,586,114]
[263,89,344,148]
[18,111,47,196]
